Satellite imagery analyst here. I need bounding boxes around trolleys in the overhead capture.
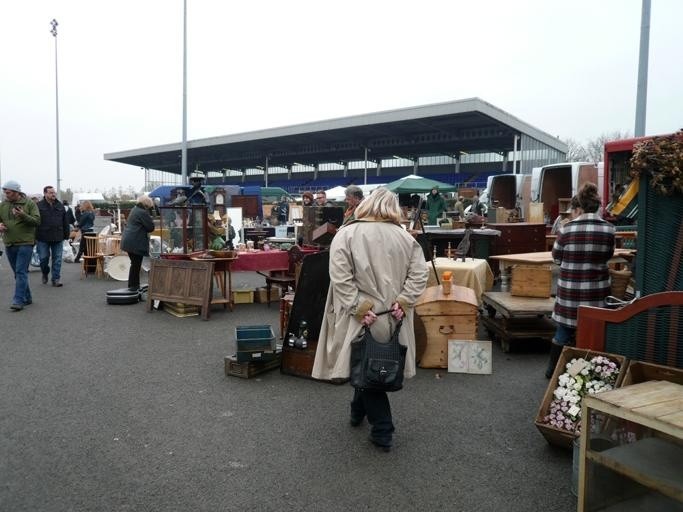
[483,289,557,321]
[479,314,560,352]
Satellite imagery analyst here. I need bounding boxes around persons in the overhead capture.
[0,181,96,311]
[301,192,319,206]
[473,196,487,216]
[121,195,155,290]
[544,182,616,381]
[315,190,333,207]
[271,195,289,225]
[341,184,367,224]
[311,187,430,451]
[454,196,465,219]
[426,186,448,224]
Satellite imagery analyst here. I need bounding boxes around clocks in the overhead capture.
[210,188,227,218]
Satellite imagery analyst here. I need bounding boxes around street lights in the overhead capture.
[50,17,63,201]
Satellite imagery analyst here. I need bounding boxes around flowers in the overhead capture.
[544,353,613,434]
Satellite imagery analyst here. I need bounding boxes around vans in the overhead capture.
[238,186,296,206]
[148,185,240,208]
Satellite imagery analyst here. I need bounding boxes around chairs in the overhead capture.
[265,244,305,308]
[195,170,512,190]
[99,234,121,278]
[80,235,103,278]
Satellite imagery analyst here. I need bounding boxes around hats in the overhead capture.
[302,191,314,201]
[2,180,20,193]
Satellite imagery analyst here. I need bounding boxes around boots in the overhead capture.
[546,336,574,379]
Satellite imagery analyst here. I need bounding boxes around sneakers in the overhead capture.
[53,279,63,287]
[367,433,393,447]
[42,267,50,284]
[351,416,364,426]
[10,300,32,310]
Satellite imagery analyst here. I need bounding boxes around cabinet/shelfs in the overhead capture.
[191,255,240,313]
[231,194,259,220]
[488,222,547,254]
[158,205,209,259]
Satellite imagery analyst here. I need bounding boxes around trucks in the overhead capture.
[462,162,604,225]
[71,192,105,206]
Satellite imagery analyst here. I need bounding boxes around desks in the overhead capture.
[481,292,555,355]
[425,256,494,303]
[489,249,637,292]
[160,249,287,311]
[574,377,683,512]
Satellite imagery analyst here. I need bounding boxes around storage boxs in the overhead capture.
[255,285,278,304]
[510,262,558,299]
[224,344,283,378]
[532,343,624,452]
[621,358,683,387]
[235,326,276,364]
[416,285,480,370]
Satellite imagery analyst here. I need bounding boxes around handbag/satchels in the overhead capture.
[349,329,407,390]
